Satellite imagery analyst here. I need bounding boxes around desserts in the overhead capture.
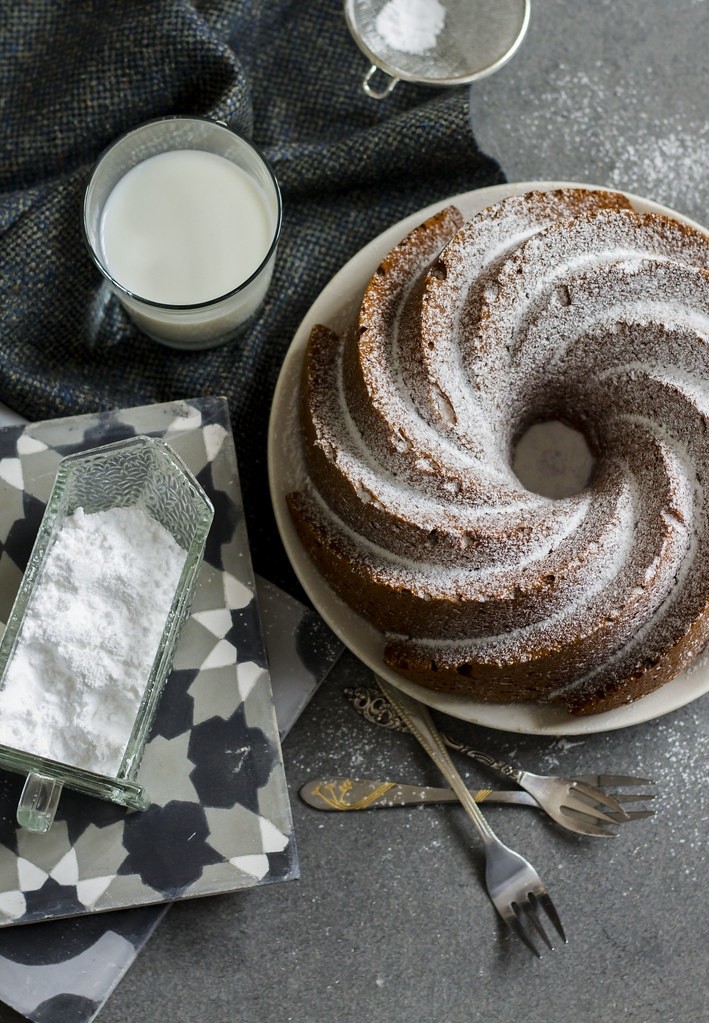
[284,186,709,715]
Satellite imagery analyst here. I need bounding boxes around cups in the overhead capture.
[79,116,283,354]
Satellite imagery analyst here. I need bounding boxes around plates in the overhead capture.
[0,390,301,928]
[264,182,709,739]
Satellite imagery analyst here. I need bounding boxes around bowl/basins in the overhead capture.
[341,0,533,100]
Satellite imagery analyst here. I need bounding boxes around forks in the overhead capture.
[302,673,656,961]
